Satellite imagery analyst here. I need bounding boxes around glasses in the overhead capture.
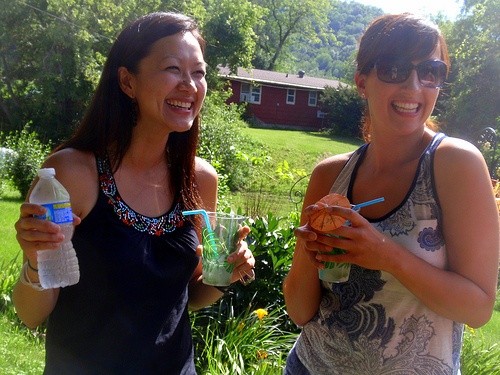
[373,56,448,88]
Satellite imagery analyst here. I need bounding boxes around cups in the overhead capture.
[201,211,245,287]
[318,204,359,283]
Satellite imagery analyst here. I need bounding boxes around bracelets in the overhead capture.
[19,259,46,291]
[214,283,231,292]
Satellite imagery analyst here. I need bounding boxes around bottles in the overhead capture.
[29,169,79,289]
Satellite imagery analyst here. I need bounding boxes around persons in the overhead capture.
[13,11,256,375]
[282,13,500,375]
